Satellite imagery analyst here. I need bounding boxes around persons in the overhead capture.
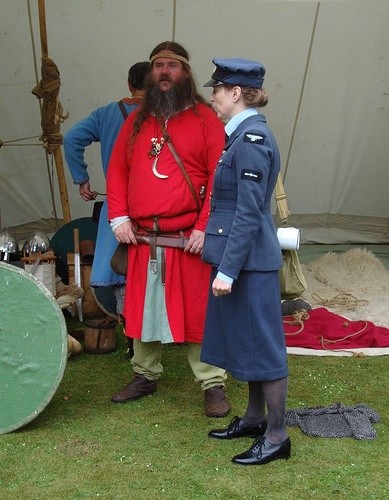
[201,57,292,465]
[105,41,232,416]
[64,61,150,358]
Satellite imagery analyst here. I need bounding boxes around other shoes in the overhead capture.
[112,376,157,402]
[204,385,230,417]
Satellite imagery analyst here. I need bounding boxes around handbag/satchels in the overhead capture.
[278,249,307,300]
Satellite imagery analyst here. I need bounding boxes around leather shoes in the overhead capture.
[232,432,292,466]
[208,415,267,440]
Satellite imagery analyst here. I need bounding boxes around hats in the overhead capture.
[202,58,265,89]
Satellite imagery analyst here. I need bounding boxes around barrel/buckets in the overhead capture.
[83,319,117,353]
[67,252,99,316]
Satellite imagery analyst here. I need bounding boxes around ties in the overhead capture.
[225,133,230,144]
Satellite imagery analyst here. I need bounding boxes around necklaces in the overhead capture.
[151,115,170,178]
